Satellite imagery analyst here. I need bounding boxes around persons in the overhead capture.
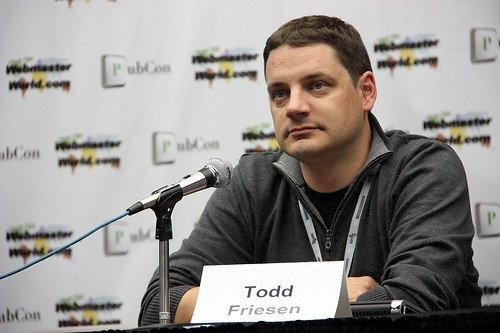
[138,13,483,327]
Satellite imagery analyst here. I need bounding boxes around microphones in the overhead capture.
[127,158,234,215]
